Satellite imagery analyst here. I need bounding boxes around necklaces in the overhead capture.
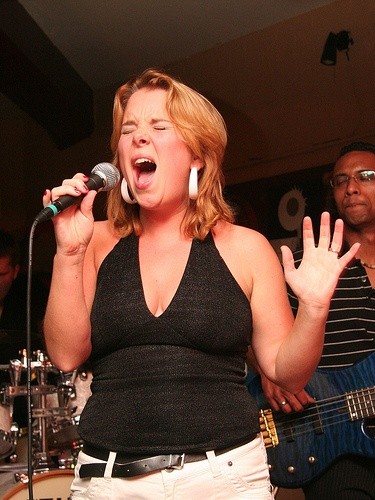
[358,257,375,270]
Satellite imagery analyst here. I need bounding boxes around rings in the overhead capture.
[328,248,340,255]
[278,402,287,407]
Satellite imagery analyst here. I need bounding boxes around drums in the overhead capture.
[1,381,15,461]
[33,414,91,471]
[32,369,93,435]
[1,363,66,436]
[0,469,77,500]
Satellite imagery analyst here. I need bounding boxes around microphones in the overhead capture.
[35,162,120,223]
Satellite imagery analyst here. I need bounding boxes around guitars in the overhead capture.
[245,351,375,489]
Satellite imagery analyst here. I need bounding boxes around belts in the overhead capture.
[78,430,259,477]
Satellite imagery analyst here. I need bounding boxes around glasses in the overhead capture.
[330,170,375,191]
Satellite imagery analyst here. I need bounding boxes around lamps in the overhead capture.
[320,30,354,65]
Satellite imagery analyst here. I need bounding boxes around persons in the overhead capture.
[245,141,375,500]
[0,230,54,427]
[43,67,361,500]
